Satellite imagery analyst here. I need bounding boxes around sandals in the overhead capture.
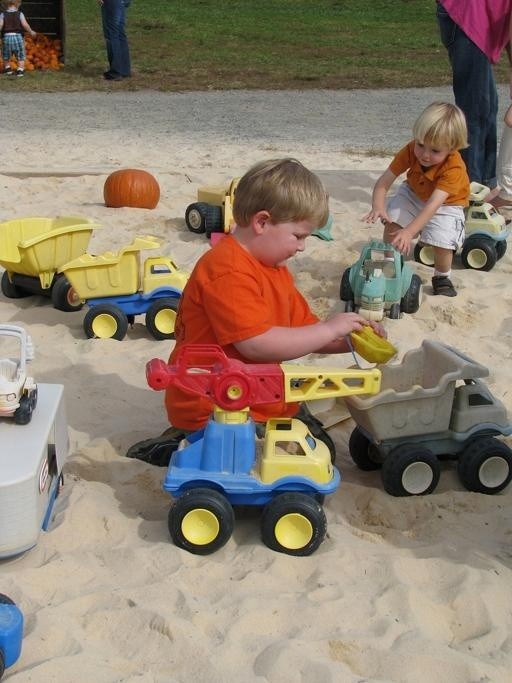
[125,425,187,468]
[431,275,457,297]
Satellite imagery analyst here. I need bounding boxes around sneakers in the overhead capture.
[2,68,13,75]
[103,70,123,81]
[15,68,24,77]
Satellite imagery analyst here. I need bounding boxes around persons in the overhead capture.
[96,0,132,81]
[360,102,470,297]
[436,0,512,202]
[488,104,512,210]
[126,158,388,467]
[0,0,37,77]
